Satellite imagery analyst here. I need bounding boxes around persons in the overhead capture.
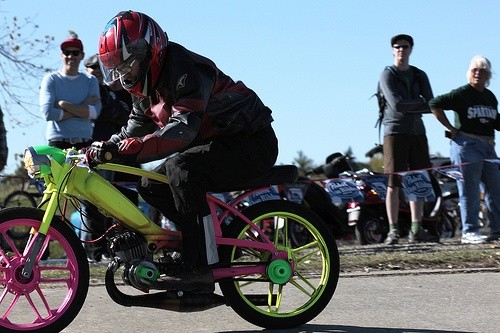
[84,53,140,247]
[0,105,9,171]
[379,34,439,245]
[40,29,111,264]
[429,55,500,243]
[83,11,279,295]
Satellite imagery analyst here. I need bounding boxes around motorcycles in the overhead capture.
[0,141,344,333]
[71,146,491,263]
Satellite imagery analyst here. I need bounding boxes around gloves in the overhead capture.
[86,141,118,165]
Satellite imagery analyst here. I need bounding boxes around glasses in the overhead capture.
[395,45,408,49]
[116,60,136,74]
[471,68,486,74]
[63,50,84,56]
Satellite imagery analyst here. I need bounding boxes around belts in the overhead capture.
[50,138,90,144]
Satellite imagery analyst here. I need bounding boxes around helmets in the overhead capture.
[98,10,168,98]
[85,55,99,67]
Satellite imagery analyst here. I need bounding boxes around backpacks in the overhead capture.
[377,66,400,121]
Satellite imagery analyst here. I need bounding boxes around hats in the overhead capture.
[60,38,83,51]
[391,35,413,46]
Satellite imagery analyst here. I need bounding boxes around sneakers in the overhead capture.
[461,233,482,244]
[165,267,215,292]
[488,233,498,240]
[384,229,401,245]
[408,229,438,243]
